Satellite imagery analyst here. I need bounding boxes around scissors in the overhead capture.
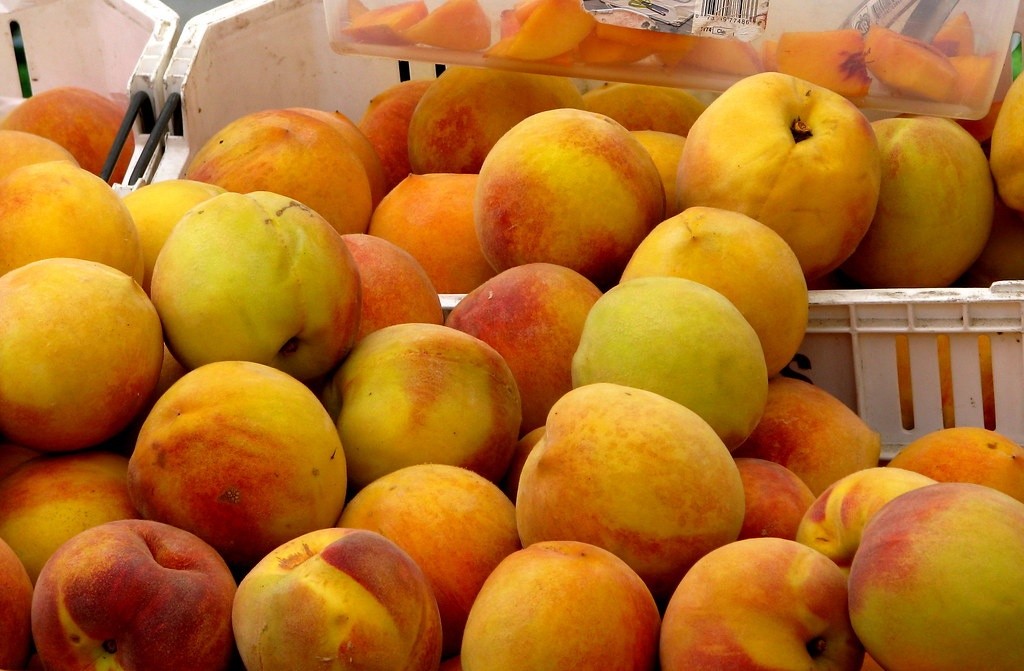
[628,0,670,17]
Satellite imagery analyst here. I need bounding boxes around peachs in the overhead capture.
[0,0,1023,671]
